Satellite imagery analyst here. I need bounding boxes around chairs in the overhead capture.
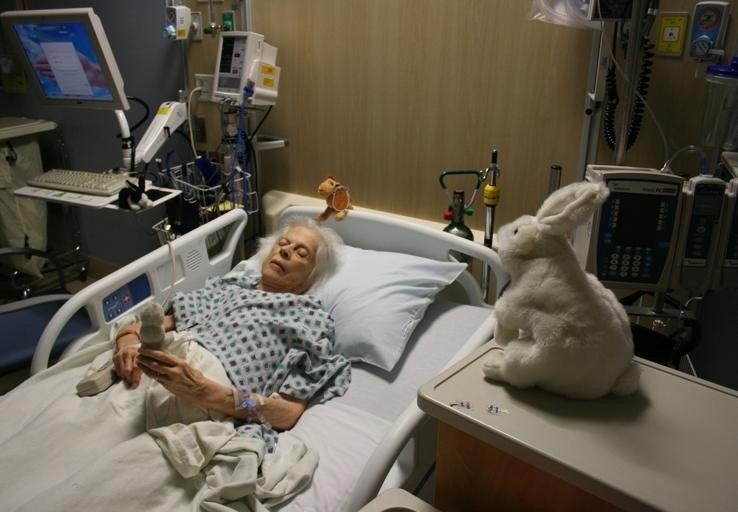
[0,244,96,374]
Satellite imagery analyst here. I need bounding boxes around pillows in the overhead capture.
[222,231,468,372]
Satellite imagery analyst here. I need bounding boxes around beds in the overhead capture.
[1,197,498,512]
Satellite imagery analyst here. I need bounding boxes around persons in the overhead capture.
[113,215,351,431]
[34,51,109,91]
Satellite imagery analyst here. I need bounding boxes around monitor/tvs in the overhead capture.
[2,8,129,112]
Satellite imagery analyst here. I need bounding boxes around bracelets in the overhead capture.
[116,329,140,342]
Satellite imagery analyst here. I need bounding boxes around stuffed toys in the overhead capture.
[137,301,174,351]
[318,180,354,222]
[484,182,639,398]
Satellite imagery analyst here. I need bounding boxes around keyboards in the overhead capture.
[26,167,132,199]
